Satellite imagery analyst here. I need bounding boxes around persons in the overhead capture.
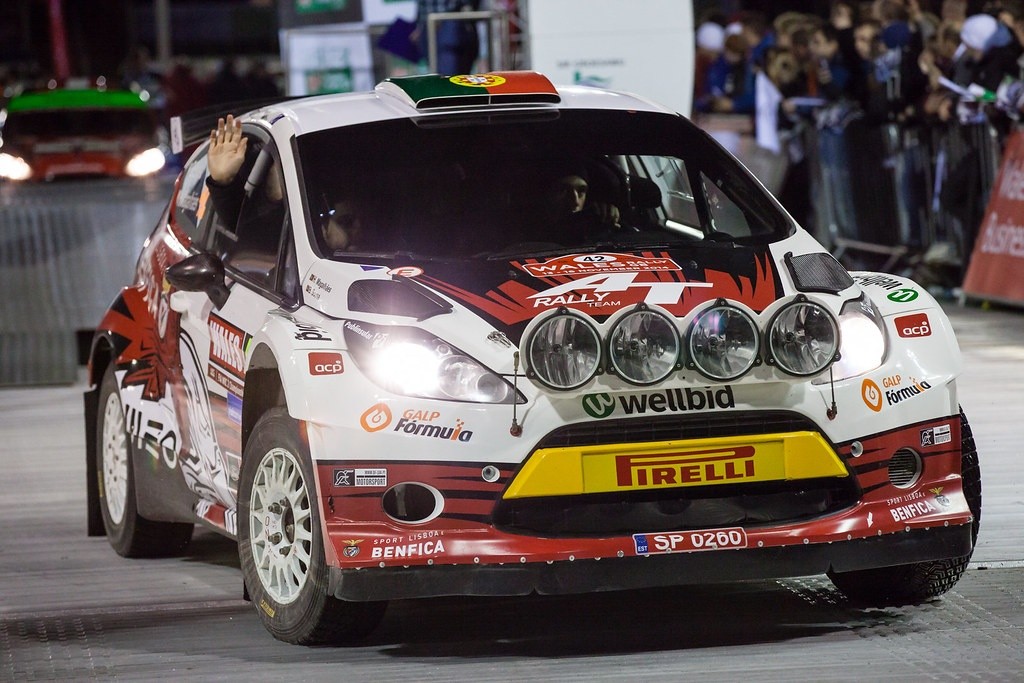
[204,114,408,257]
[513,159,629,245]
[691,0,1024,300]
[0,32,288,171]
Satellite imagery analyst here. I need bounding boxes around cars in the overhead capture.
[0,91,175,241]
[82,68,982,650]
